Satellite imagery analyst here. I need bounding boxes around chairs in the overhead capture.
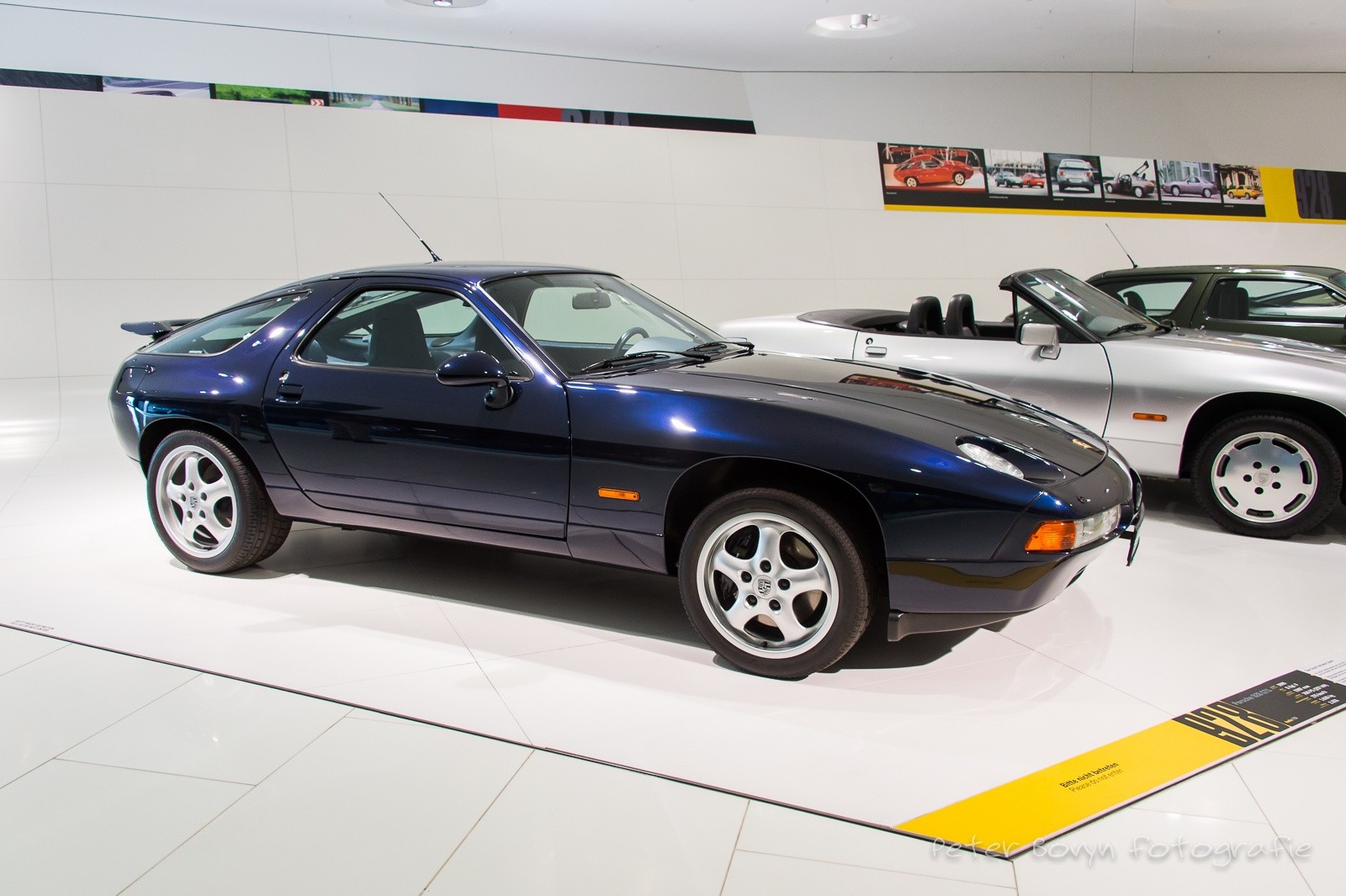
[365,302,437,371]
[473,294,532,375]
[1123,291,1146,315]
[1208,285,1237,321]
[905,296,947,337]
[944,293,982,338]
[1232,287,1249,320]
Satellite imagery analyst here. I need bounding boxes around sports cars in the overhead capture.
[1162,175,1218,198]
[893,154,973,188]
[1227,185,1260,200]
[714,267,1346,538]
[108,193,1146,682]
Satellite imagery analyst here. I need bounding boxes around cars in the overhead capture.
[1002,223,1346,351]
[1055,158,1095,193]
[995,170,1044,188]
[1103,161,1154,197]
